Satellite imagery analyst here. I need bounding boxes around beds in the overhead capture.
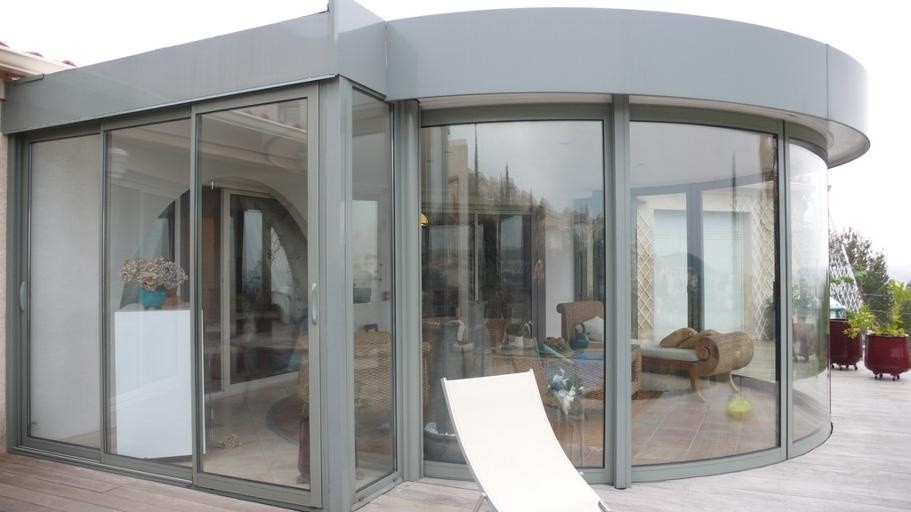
[556,300,755,404]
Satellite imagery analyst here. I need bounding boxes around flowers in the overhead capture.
[119,257,187,291]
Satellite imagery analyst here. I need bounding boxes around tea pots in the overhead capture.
[567,321,592,351]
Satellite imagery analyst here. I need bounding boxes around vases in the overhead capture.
[139,286,168,308]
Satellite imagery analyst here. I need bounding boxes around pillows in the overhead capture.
[659,327,722,348]
[575,315,603,341]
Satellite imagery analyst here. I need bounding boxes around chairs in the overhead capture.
[294,329,430,483]
[441,370,613,511]
[422,317,511,413]
[469,335,605,468]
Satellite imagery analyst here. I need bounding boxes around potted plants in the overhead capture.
[791,280,817,362]
[842,279,911,381]
[827,272,865,371]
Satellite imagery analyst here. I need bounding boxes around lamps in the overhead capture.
[420,213,428,227]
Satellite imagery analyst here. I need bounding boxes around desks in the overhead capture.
[229,330,309,445]
